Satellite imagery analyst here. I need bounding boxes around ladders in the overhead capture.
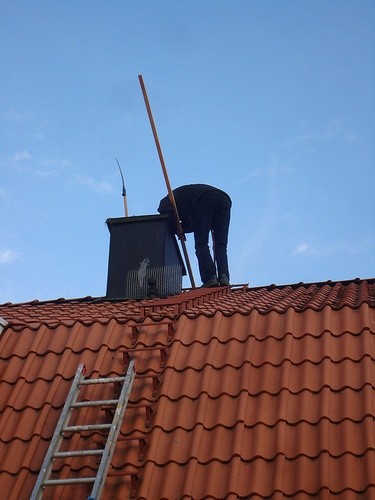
[30,360,137,499]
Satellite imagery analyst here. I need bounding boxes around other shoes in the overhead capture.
[219,273,229,286]
[201,275,219,288]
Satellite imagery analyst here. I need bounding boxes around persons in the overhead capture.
[155,183,232,287]
